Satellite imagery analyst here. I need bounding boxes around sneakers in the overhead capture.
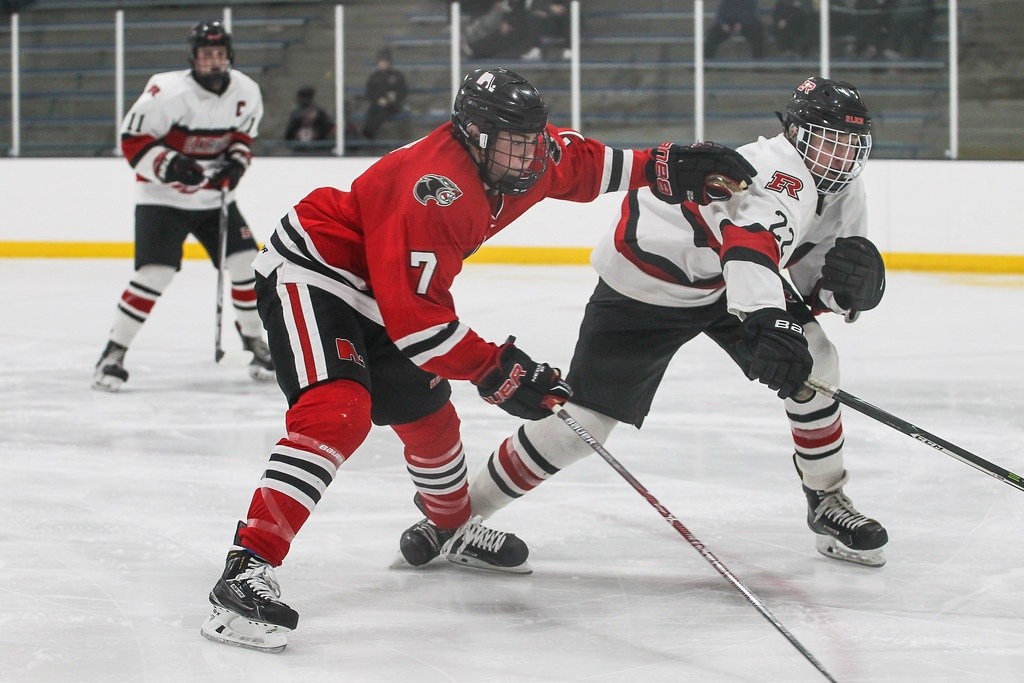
[249,340,279,382]
[389,517,454,567]
[793,455,888,568]
[93,342,129,393]
[199,520,300,653]
[415,491,532,575]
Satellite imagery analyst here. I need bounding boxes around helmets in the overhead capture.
[775,75,873,195]
[189,18,235,82]
[451,67,551,195]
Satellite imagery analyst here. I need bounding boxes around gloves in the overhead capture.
[477,334,574,422]
[645,143,758,208]
[164,156,205,185]
[826,236,886,322]
[209,162,244,192]
[741,308,813,399]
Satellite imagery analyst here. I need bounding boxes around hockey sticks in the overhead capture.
[214,178,256,370]
[550,401,837,683]
[802,374,1024,493]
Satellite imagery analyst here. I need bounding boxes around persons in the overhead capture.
[93,21,275,390]
[284,88,332,151]
[202,66,757,654]
[362,48,407,141]
[391,75,889,571]
[704,0,939,74]
[462,0,587,62]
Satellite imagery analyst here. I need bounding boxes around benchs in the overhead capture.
[1,0,1024,163]
